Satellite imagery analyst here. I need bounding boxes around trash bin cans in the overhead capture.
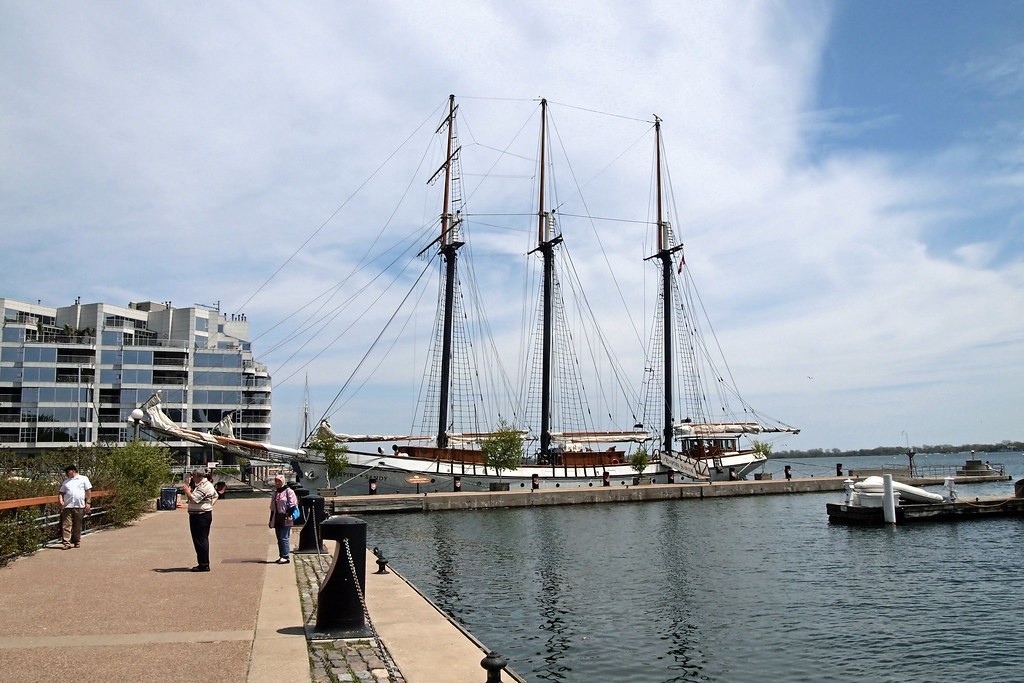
[161,488,178,510]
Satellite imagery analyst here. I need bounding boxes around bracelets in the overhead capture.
[87,502,91,504]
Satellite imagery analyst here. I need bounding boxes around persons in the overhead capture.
[182,467,219,571]
[269,475,299,564]
[58,463,93,549]
[570,445,616,452]
[985,461,993,470]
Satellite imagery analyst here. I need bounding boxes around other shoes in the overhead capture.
[63,545,70,550]
[276,557,290,564]
[192,564,210,572]
[75,544,80,548]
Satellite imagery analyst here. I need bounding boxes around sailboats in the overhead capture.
[125,90,802,495]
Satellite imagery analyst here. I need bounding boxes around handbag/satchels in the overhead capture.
[292,505,300,521]
[274,513,285,529]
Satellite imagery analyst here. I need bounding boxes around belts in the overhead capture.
[189,512,206,514]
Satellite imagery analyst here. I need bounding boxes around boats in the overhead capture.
[852,474,944,504]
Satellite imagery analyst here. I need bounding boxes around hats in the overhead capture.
[64,465,76,471]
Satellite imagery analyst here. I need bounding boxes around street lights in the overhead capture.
[132,407,145,451]
